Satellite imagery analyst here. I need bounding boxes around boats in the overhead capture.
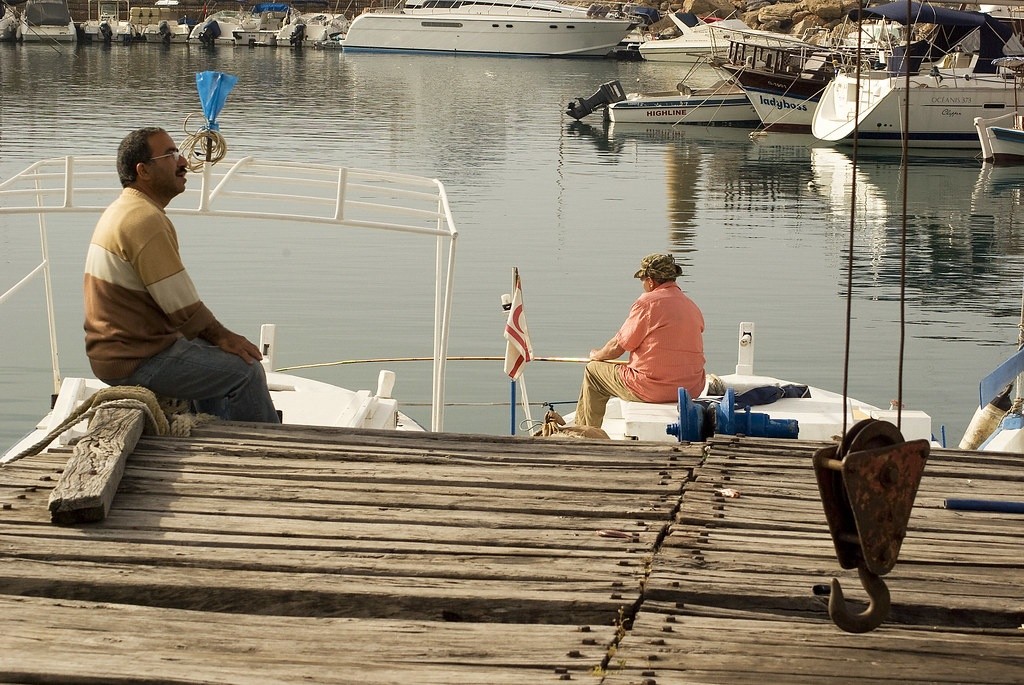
[1,1,352,50]
[567,0,1024,161]
[639,7,821,65]
[987,162,1023,184]
[338,0,643,62]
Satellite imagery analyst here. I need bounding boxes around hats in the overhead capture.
[634,254,682,279]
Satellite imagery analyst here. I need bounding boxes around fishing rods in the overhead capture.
[275,356,630,374]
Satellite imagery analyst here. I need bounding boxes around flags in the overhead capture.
[504,275,534,380]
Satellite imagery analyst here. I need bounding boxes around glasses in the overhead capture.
[149,151,182,160]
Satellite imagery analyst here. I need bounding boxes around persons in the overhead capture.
[574,254,706,428]
[84,126,280,422]
